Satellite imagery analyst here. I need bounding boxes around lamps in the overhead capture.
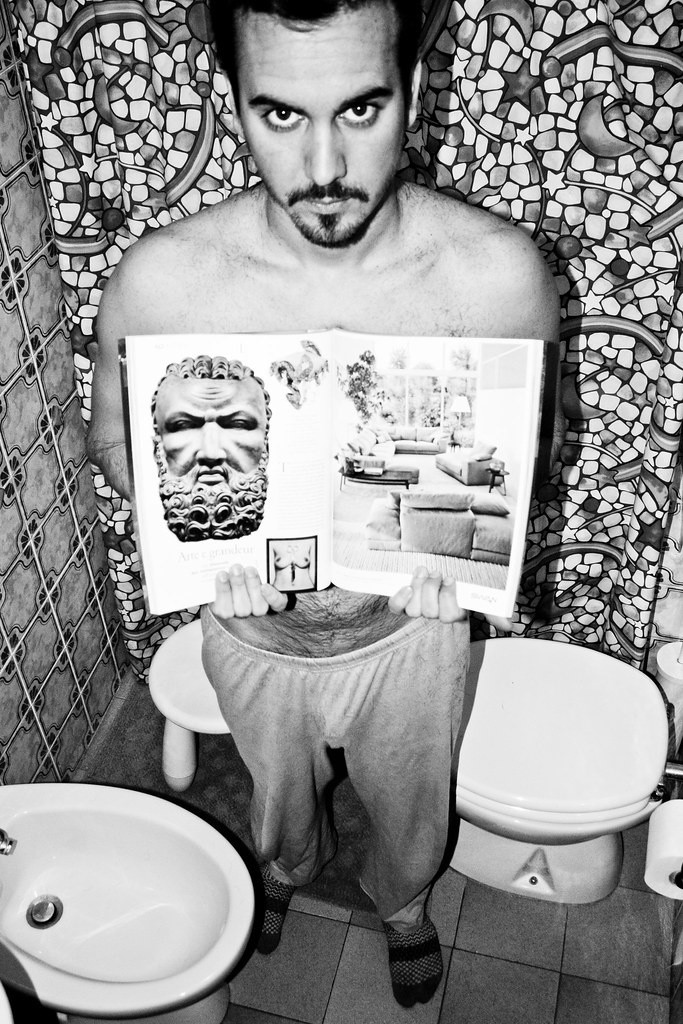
[450,396,472,430]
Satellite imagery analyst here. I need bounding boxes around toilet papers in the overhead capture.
[644,799,683,901]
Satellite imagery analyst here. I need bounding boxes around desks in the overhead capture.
[339,466,412,490]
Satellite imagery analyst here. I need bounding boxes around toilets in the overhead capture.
[445,636,670,905]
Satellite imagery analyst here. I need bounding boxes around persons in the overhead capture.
[151,354,271,541]
[92,0,556,1009]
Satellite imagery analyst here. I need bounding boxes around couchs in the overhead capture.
[435,447,505,486]
[335,423,447,484]
[333,491,513,590]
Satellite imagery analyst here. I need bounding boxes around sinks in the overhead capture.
[0,782,257,1024]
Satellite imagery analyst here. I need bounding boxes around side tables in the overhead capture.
[486,468,510,496]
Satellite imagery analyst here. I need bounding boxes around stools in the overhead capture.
[149,618,231,792]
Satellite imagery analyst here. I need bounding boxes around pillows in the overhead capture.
[429,430,447,443]
[359,444,370,456]
[474,454,492,461]
[376,435,386,444]
[471,495,511,516]
